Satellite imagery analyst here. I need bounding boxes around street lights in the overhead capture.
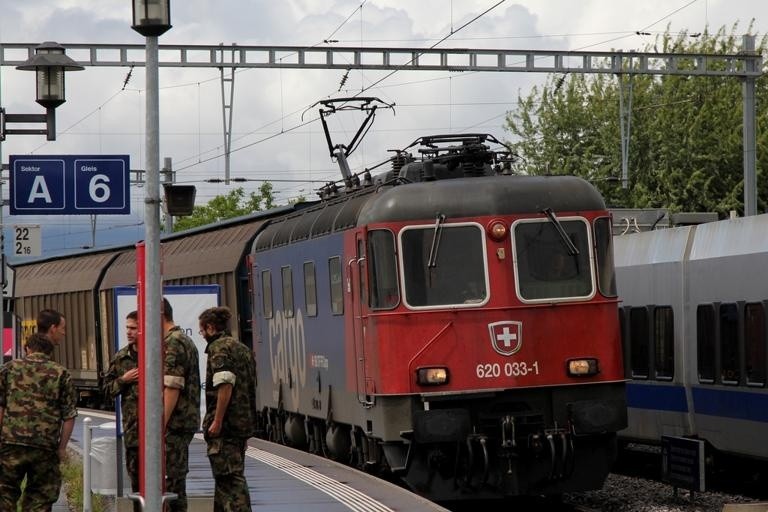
[121,2,173,511]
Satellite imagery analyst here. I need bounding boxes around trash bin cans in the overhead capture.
[83,416,132,497]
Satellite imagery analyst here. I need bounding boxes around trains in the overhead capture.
[1,131,626,501]
[608,203,767,490]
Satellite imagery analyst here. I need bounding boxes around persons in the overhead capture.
[107,310,139,511]
[0,332,78,512]
[34,309,68,346]
[160,296,202,511]
[197,305,254,511]
